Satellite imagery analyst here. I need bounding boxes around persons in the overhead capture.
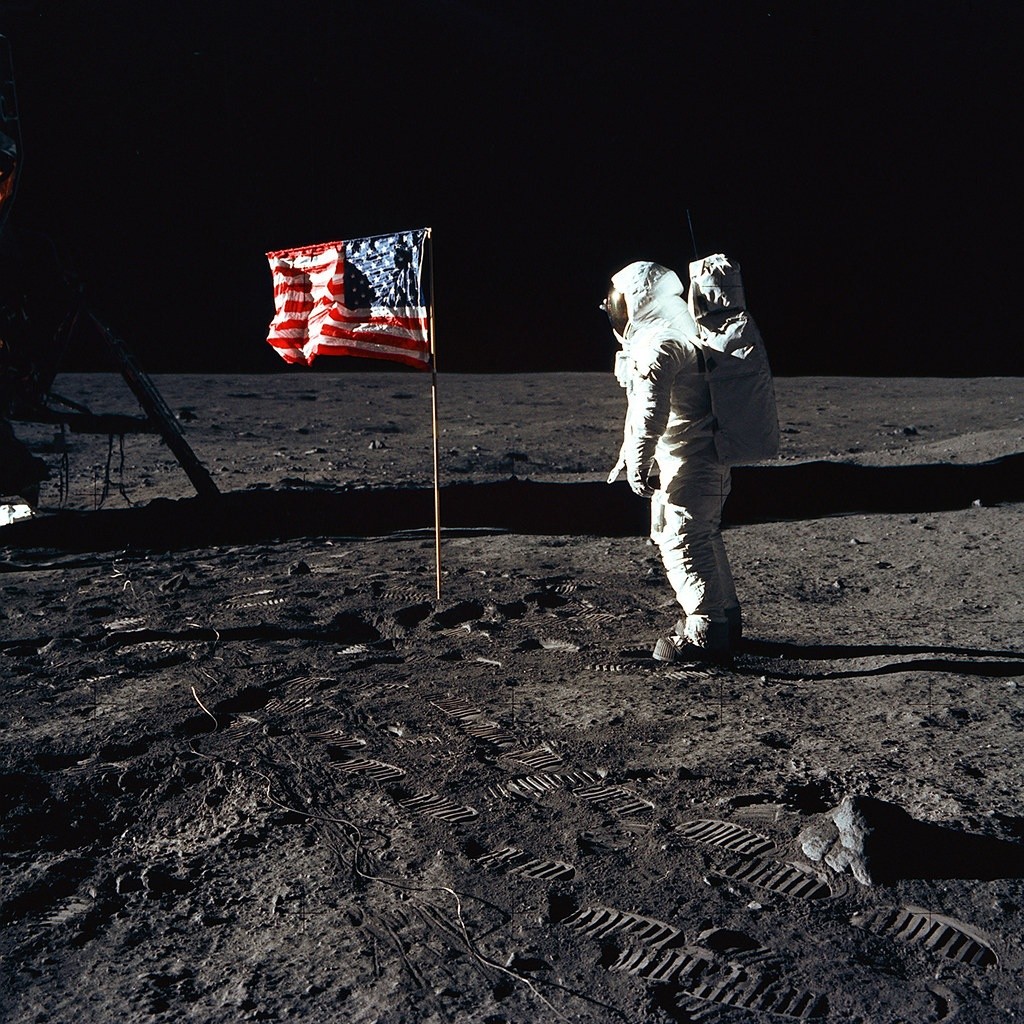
[606,261,742,663]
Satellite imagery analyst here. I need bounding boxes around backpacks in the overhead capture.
[639,254,779,463]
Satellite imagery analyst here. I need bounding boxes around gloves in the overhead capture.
[627,466,654,499]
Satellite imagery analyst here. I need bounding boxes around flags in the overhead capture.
[266,228,432,374]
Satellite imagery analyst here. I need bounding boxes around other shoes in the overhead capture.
[674,610,743,639]
[652,635,727,663]
